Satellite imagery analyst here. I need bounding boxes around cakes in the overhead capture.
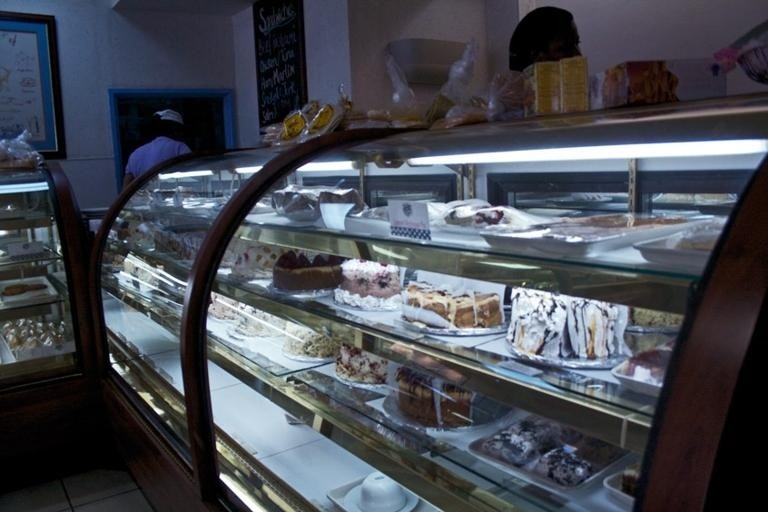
[231,244,305,280]
[180,232,242,268]
[209,290,239,320]
[235,302,285,338]
[2,283,49,302]
[272,249,346,289]
[506,285,631,361]
[482,414,568,466]
[153,226,208,259]
[282,319,341,359]
[6,241,45,261]
[402,280,502,326]
[335,343,389,385]
[393,364,513,427]
[333,258,405,308]
[531,447,590,483]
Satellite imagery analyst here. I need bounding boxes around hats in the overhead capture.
[154,109,183,124]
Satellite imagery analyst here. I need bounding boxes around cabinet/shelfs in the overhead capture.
[0,160,92,492]
[89,92,768,512]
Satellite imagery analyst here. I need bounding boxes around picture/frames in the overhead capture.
[0,11,66,159]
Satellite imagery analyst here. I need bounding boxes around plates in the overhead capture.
[346,483,419,512]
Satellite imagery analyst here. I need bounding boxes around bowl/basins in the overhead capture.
[356,470,406,512]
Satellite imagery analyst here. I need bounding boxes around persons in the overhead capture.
[123,109,193,187]
